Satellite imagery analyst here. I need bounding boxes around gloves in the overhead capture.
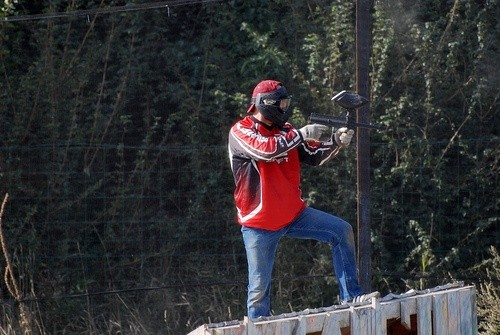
[334,126,355,149]
[299,123,329,142]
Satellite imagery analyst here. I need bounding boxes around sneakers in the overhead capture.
[342,290,380,305]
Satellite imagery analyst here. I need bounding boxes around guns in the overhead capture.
[309,90,381,132]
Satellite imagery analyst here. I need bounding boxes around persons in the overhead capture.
[228,81,381,320]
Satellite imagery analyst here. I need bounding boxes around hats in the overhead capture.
[246,80,281,113]
